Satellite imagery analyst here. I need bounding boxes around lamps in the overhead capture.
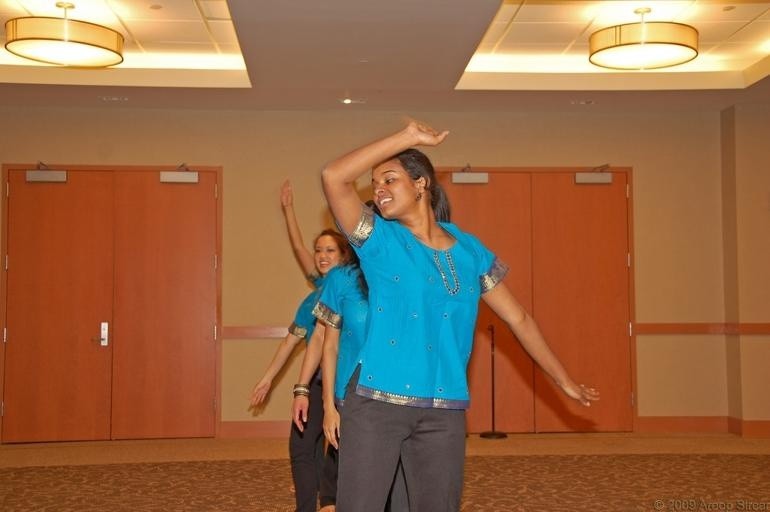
[4,2,129,70]
[582,4,702,73]
